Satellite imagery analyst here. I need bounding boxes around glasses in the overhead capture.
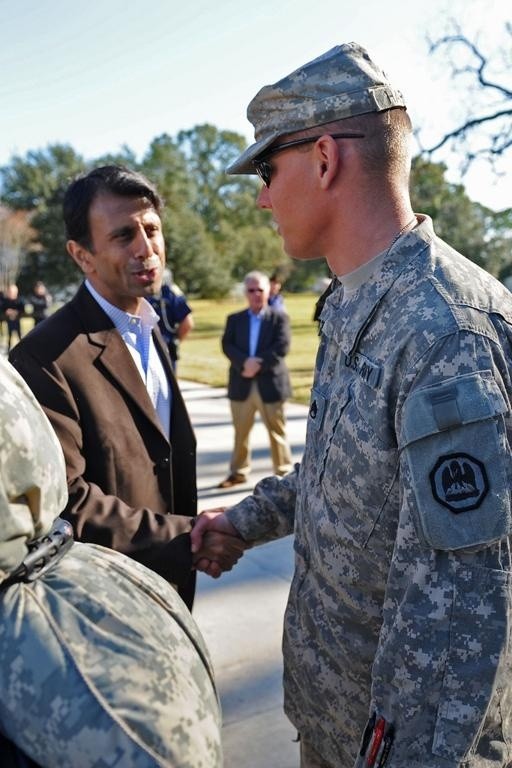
[251,133,365,186]
[248,289,264,292]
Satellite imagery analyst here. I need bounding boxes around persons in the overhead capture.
[218,269,293,488]
[0,165,249,768]
[191,41,511,768]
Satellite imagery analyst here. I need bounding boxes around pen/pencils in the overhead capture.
[360,711,396,768]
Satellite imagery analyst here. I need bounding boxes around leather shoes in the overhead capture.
[219,473,246,488]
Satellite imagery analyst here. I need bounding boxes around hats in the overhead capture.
[225,42,406,175]
